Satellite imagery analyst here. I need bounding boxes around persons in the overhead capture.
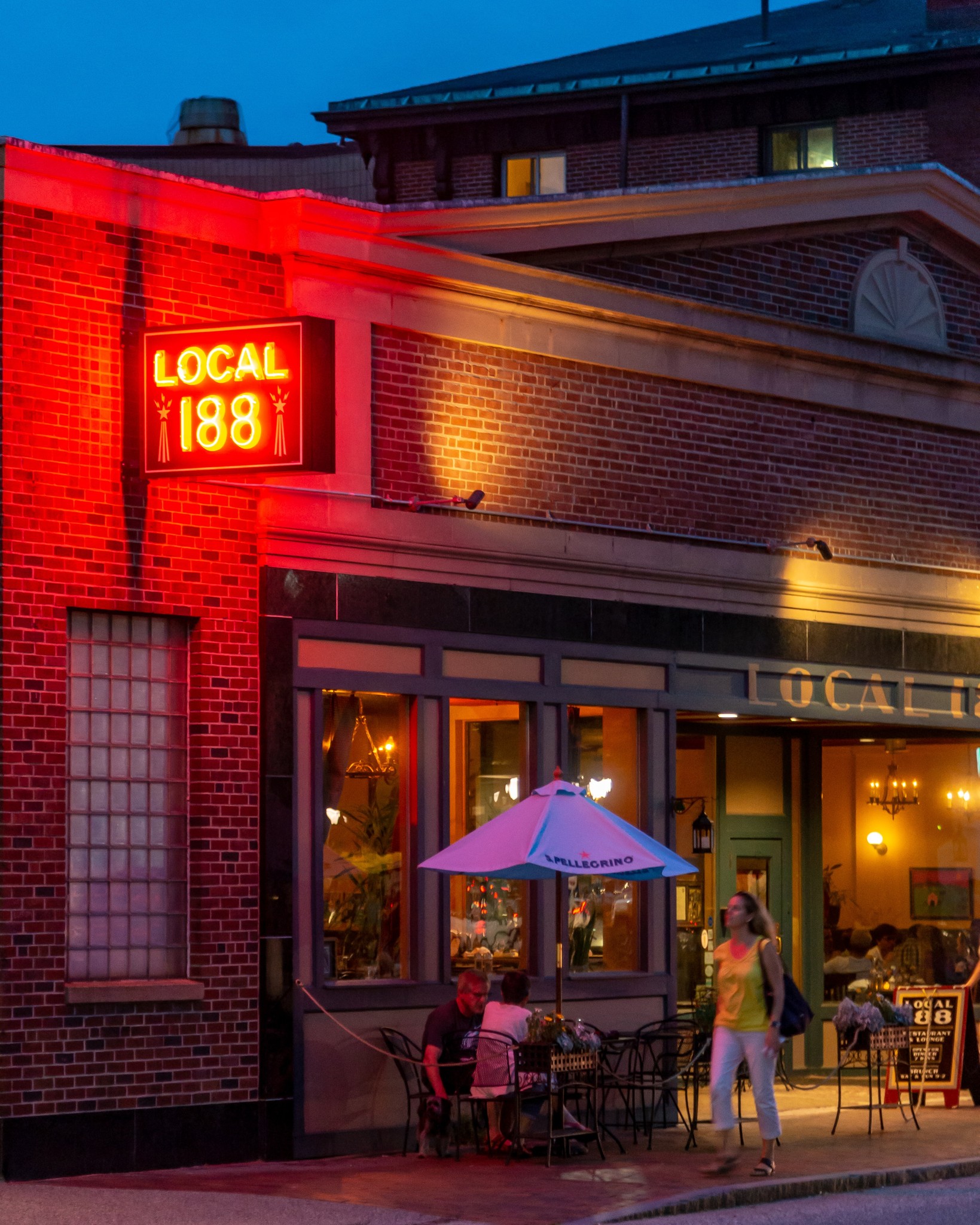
[884,922,941,986]
[366,875,604,980]
[470,969,595,1158]
[823,922,882,1006]
[420,967,548,1158]
[696,890,785,1179]
[956,918,980,1006]
[863,924,900,989]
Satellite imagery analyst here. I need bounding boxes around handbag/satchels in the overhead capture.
[758,938,812,1036]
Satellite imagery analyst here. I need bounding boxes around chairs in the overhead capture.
[564,1016,707,1158]
[448,1028,569,1165]
[377,1027,493,1160]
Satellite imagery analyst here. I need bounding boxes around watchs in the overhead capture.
[770,1020,781,1028]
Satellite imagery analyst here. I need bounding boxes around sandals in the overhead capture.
[698,1155,742,1174]
[751,1158,776,1176]
[483,1137,514,1151]
[513,1146,533,1157]
[562,1122,592,1131]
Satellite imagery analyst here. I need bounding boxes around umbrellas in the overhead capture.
[414,764,698,1161]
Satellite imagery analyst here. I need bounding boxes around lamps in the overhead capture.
[866,740,919,820]
[866,831,887,855]
[410,490,486,512]
[670,796,715,854]
[946,743,980,820]
[344,697,396,785]
[769,537,833,560]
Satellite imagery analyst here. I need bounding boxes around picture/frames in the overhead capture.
[908,866,975,921]
